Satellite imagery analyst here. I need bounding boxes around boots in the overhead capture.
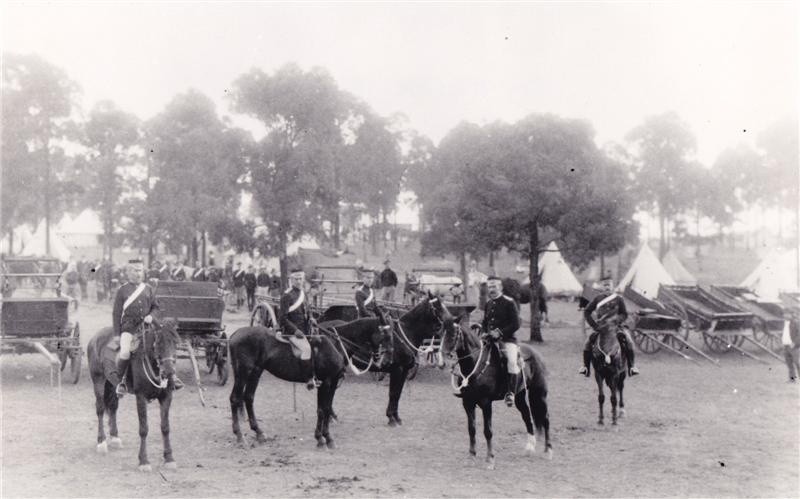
[579,350,591,376]
[625,349,639,376]
[301,359,314,389]
[506,373,517,407]
[117,358,129,398]
[175,382,183,389]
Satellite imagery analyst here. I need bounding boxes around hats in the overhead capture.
[601,277,613,284]
[362,269,374,277]
[487,276,501,287]
[128,259,144,271]
[291,268,305,278]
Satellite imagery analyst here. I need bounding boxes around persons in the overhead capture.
[111,260,183,393]
[779,306,799,380]
[478,277,522,407]
[279,268,320,390]
[355,270,387,366]
[142,256,282,310]
[307,267,328,311]
[577,278,639,376]
[0,258,127,302]
[360,258,417,305]
[538,279,548,320]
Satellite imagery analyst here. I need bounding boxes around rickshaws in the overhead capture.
[146,276,231,407]
[257,281,483,385]
[578,283,799,369]
[0,258,84,385]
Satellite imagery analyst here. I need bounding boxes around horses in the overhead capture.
[436,314,554,471]
[517,281,549,320]
[590,305,628,426]
[86,315,179,474]
[227,288,452,450]
